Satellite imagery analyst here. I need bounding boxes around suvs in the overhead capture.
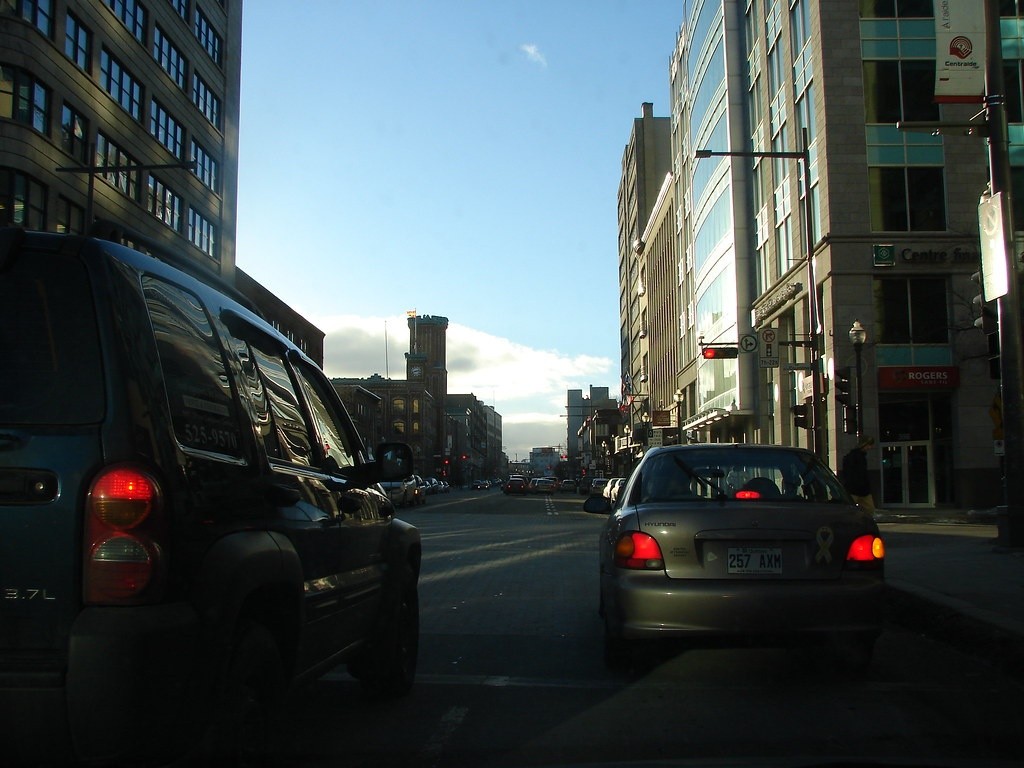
[0,225,425,768]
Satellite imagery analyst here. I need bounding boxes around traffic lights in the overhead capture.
[970,263,999,335]
[834,366,851,406]
[701,347,739,361]
[793,404,809,429]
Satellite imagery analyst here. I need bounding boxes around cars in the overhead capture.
[602,478,619,499]
[475,473,578,496]
[611,478,628,503]
[583,442,887,668]
[381,473,451,509]
[590,478,610,497]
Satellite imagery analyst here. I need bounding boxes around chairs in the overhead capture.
[743,476,782,499]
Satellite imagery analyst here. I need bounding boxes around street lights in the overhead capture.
[848,317,868,445]
[692,125,824,462]
